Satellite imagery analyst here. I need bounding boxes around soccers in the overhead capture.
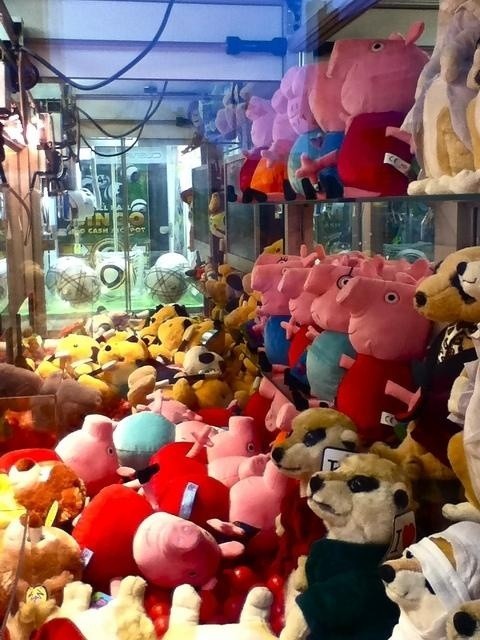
[45,256,190,309]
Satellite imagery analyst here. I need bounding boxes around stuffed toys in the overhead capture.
[0,0,480,640]
